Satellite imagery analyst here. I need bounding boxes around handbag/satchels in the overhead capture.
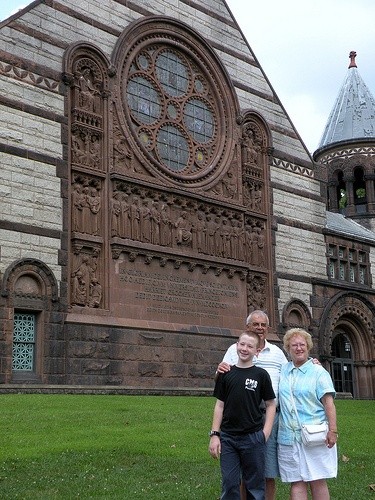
[301,423,329,447]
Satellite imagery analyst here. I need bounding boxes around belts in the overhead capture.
[263,406,280,413]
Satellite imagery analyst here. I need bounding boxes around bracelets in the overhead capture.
[329,429,338,434]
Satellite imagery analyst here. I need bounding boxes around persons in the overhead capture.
[214,310,322,500]
[209,331,276,500]
[113,136,134,172]
[242,130,262,165]
[247,283,266,315]
[242,182,262,211]
[221,171,238,200]
[71,131,102,169]
[174,211,193,246]
[72,256,102,307]
[277,328,338,500]
[196,212,264,265]
[79,68,100,113]
[111,192,172,246]
[72,184,101,236]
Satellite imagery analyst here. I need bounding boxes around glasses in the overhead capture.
[248,322,267,327]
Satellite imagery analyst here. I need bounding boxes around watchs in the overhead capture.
[209,431,220,437]
